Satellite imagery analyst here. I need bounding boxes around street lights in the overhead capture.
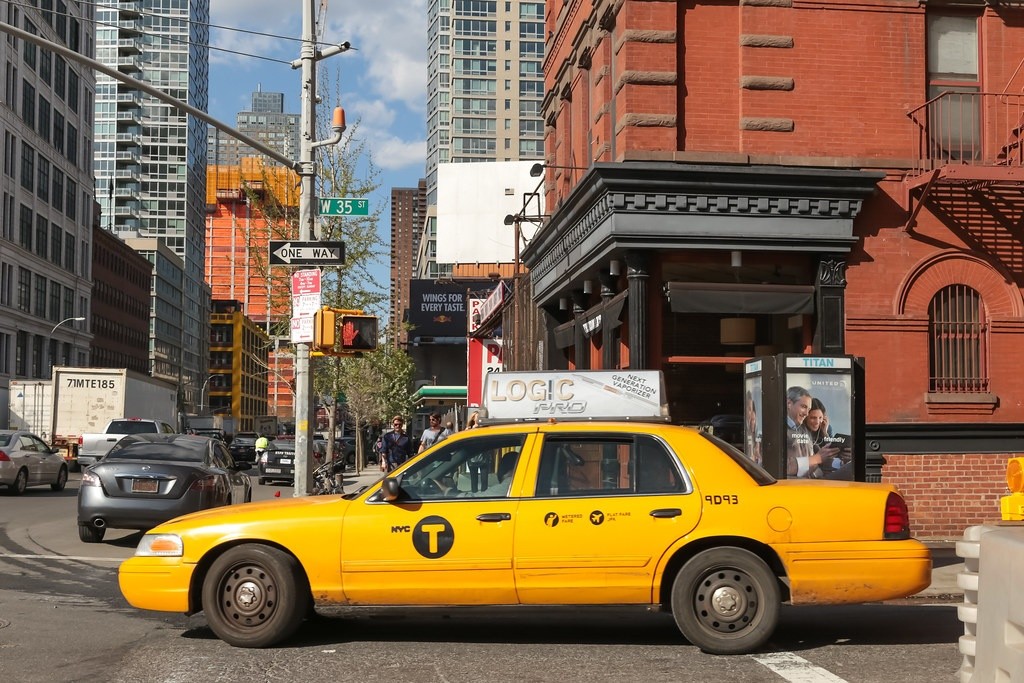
[46,317,85,380]
[200,373,224,411]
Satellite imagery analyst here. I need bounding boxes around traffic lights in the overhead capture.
[340,315,378,351]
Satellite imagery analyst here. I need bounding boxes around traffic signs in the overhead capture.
[290,317,314,344]
[291,268,322,319]
[267,240,347,267]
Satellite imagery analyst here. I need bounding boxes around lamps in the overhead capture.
[559,298,567,310]
[504,214,551,225]
[583,280,593,294]
[731,251,742,268]
[530,163,588,178]
[609,259,622,275]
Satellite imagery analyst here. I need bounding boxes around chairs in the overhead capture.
[647,451,671,488]
[540,446,570,495]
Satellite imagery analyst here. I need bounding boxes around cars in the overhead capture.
[0,429,69,494]
[77,432,252,543]
[228,434,357,485]
[120,368,933,649]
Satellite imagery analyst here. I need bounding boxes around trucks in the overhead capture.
[187,415,234,444]
[7,379,53,445]
[253,415,278,435]
[48,366,183,473]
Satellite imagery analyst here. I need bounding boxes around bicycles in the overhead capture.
[312,460,344,496]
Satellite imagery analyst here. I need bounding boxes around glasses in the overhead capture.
[394,424,402,427]
[430,418,435,421]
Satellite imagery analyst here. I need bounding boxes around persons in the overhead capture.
[381,415,414,474]
[433,451,519,498]
[373,436,382,468]
[417,413,452,454]
[787,387,852,479]
[744,390,763,468]
[255,433,270,463]
[465,412,480,431]
[467,453,491,493]
